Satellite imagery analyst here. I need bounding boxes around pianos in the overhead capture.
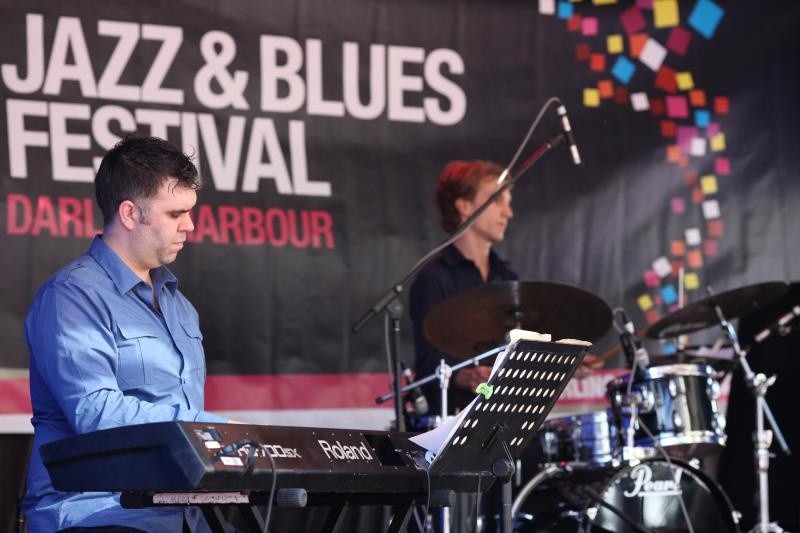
[39,420,498,533]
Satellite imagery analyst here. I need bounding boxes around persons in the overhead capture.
[24,136,267,533]
[409,160,605,533]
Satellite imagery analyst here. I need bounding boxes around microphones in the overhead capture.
[401,361,429,416]
[751,306,800,347]
[555,103,582,165]
[622,311,649,368]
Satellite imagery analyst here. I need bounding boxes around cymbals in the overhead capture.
[422,281,613,367]
[646,281,791,339]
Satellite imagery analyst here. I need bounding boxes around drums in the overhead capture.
[542,409,629,466]
[511,455,741,533]
[605,362,728,461]
